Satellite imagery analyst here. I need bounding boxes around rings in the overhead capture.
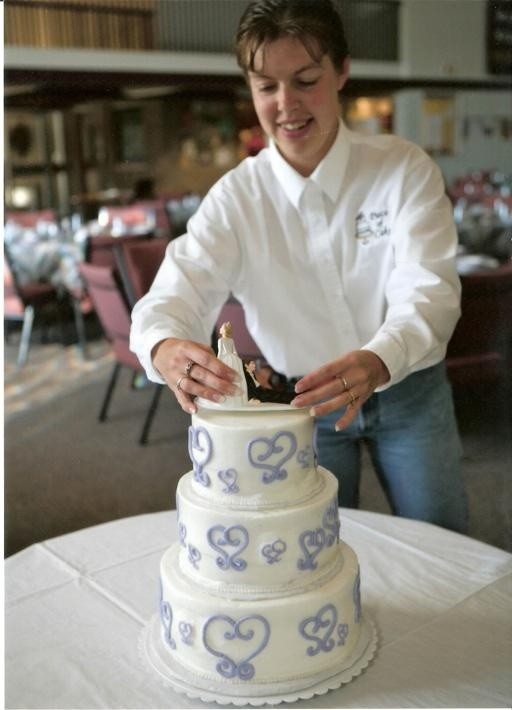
[345,389,355,407]
[175,374,189,390]
[184,360,196,376]
[336,374,348,392]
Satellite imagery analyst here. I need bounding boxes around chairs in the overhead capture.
[61,231,156,363]
[112,236,263,401]
[4,243,63,366]
[6,191,204,238]
[78,262,200,444]
[445,173,512,432]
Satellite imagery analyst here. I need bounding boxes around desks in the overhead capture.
[4,506,512,709]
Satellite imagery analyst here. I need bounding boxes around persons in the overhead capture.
[128,1,473,538]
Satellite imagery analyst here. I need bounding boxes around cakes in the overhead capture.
[157,321,360,695]
